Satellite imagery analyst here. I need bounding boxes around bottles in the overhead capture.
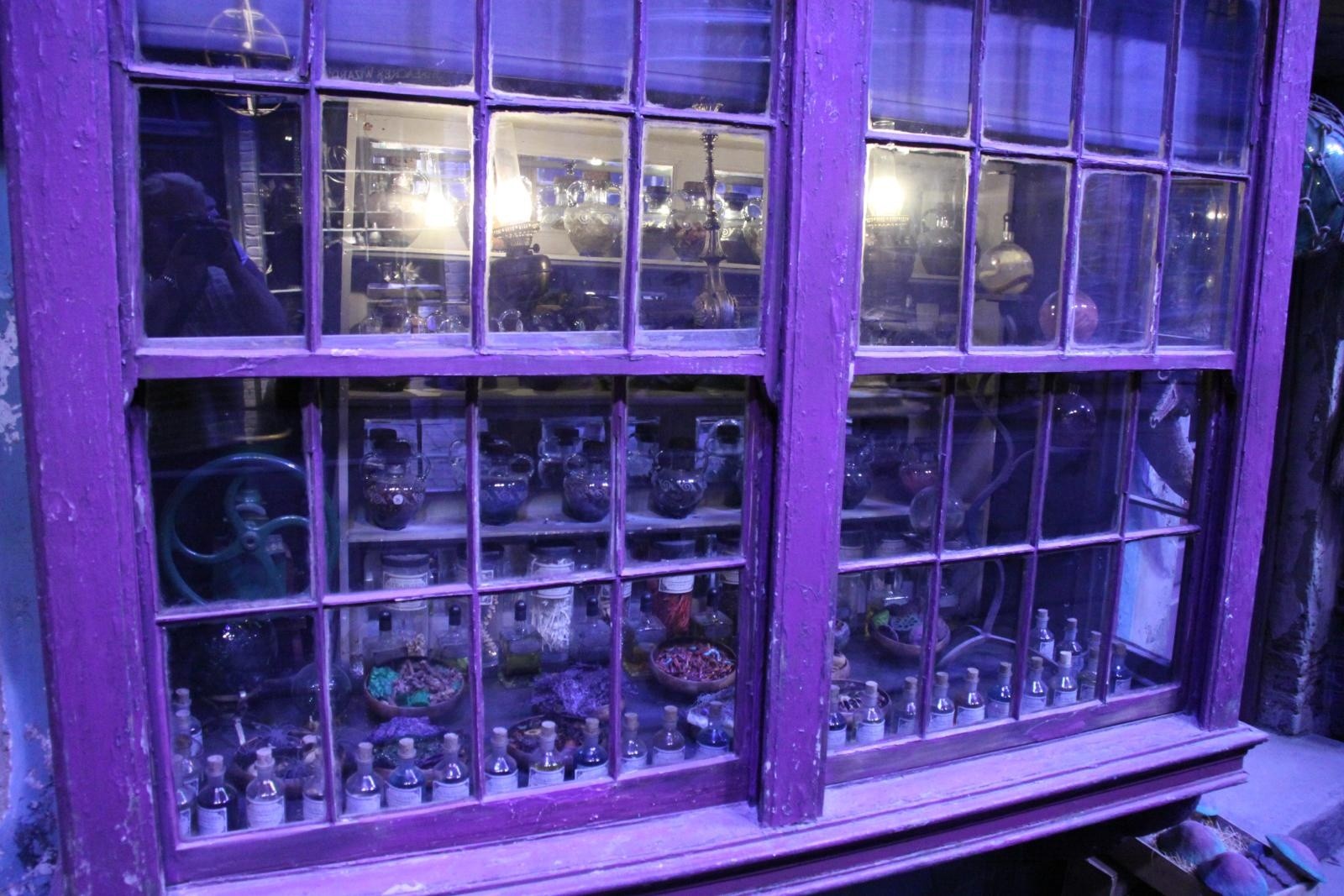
[320,151,1108,337]
[160,538,1133,837]
[351,438,948,531]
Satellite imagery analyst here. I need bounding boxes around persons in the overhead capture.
[144,170,290,620]
